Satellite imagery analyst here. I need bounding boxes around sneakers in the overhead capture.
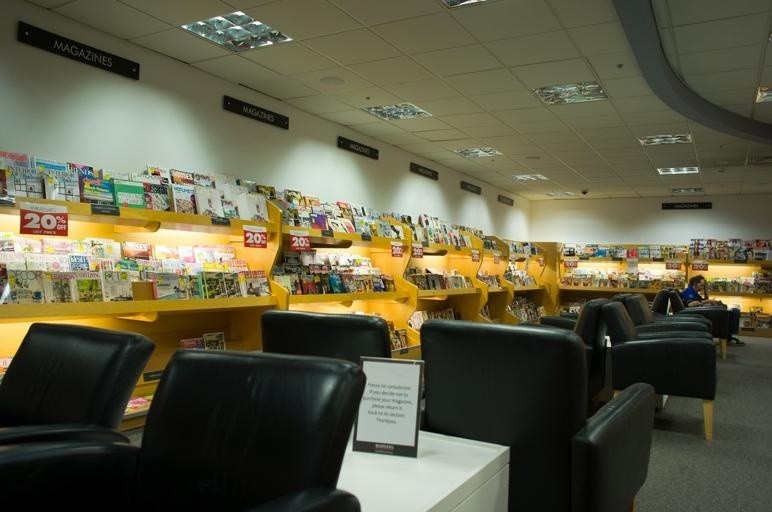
[726,337,745,346]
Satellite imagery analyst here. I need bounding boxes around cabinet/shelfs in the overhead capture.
[3,182,291,434]
[558,242,771,339]
[273,216,421,360]
[407,230,557,333]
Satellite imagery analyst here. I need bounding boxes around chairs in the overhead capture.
[0,346,363,512]
[260,309,392,361]
[1,322,156,443]
[419,318,656,511]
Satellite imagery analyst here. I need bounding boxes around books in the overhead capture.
[124,397,151,415]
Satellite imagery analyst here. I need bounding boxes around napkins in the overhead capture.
[539,286,732,441]
